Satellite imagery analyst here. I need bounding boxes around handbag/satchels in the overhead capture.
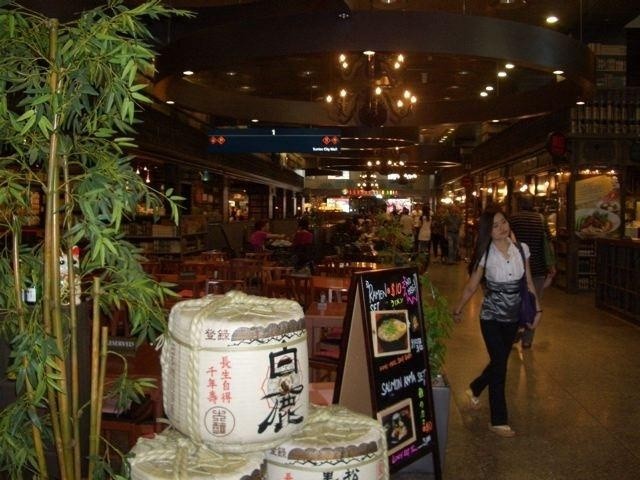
[544,239,555,267]
[520,281,535,326]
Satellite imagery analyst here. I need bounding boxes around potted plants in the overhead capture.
[376,222,452,477]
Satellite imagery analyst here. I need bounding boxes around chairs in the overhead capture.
[69,216,411,470]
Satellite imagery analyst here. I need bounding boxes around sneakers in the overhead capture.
[487,421,516,438]
[465,389,480,409]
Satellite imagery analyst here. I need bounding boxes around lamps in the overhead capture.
[323,51,422,133]
[357,170,380,191]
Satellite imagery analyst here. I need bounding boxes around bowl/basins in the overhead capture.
[376,313,407,350]
[575,207,622,237]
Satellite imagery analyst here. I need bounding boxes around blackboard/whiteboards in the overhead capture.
[331,273,370,417]
[363,268,434,474]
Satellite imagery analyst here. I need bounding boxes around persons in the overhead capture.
[452,204,544,439]
[505,198,558,349]
[249,219,285,249]
[390,202,450,269]
[350,213,381,236]
[292,221,313,245]
